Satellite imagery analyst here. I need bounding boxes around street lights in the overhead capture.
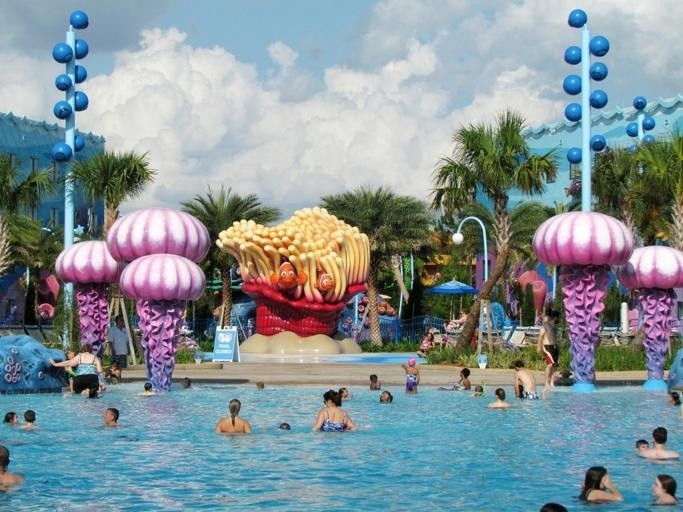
[47,12,94,348]
[451,216,493,367]
[622,94,658,184]
[556,10,615,214]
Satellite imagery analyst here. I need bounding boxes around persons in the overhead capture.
[49,314,191,398]
[669,391,682,407]
[215,388,356,437]
[539,424,683,511]
[0,408,120,482]
[338,312,559,408]
